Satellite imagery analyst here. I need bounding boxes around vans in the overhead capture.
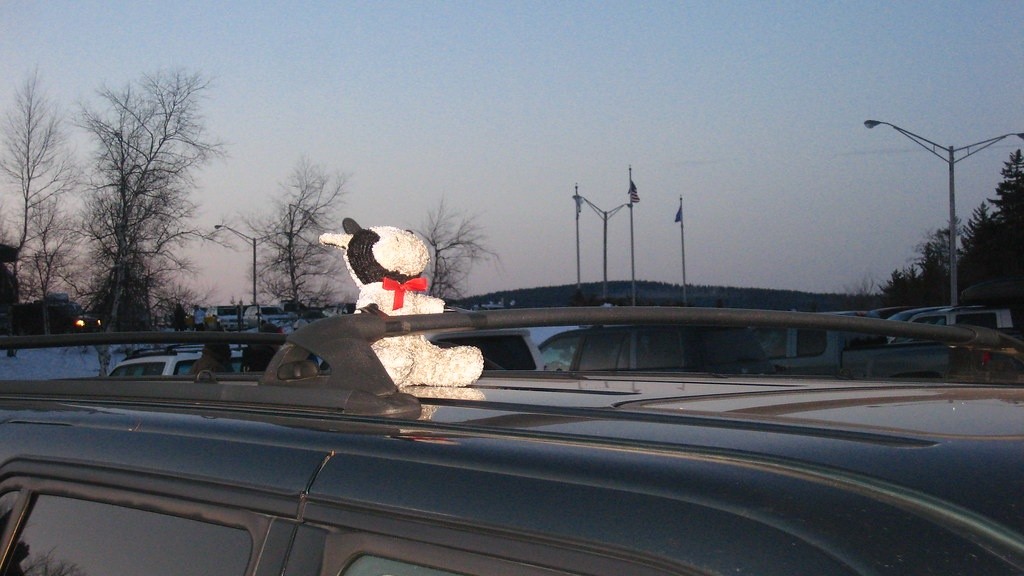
[204,306,242,330]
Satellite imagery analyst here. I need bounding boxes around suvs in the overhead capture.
[242,305,292,328]
[106,345,243,378]
[0,300,1024,575]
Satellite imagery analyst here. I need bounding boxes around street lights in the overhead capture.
[572,192,633,298]
[214,223,291,303]
[862,116,1024,305]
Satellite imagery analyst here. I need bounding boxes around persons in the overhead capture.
[194,305,206,331]
[174,303,186,331]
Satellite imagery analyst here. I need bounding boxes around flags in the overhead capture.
[674,206,683,223]
[629,181,640,203]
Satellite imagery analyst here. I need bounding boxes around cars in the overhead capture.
[750,303,1024,378]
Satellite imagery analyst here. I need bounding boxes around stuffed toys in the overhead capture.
[320,217,484,388]
